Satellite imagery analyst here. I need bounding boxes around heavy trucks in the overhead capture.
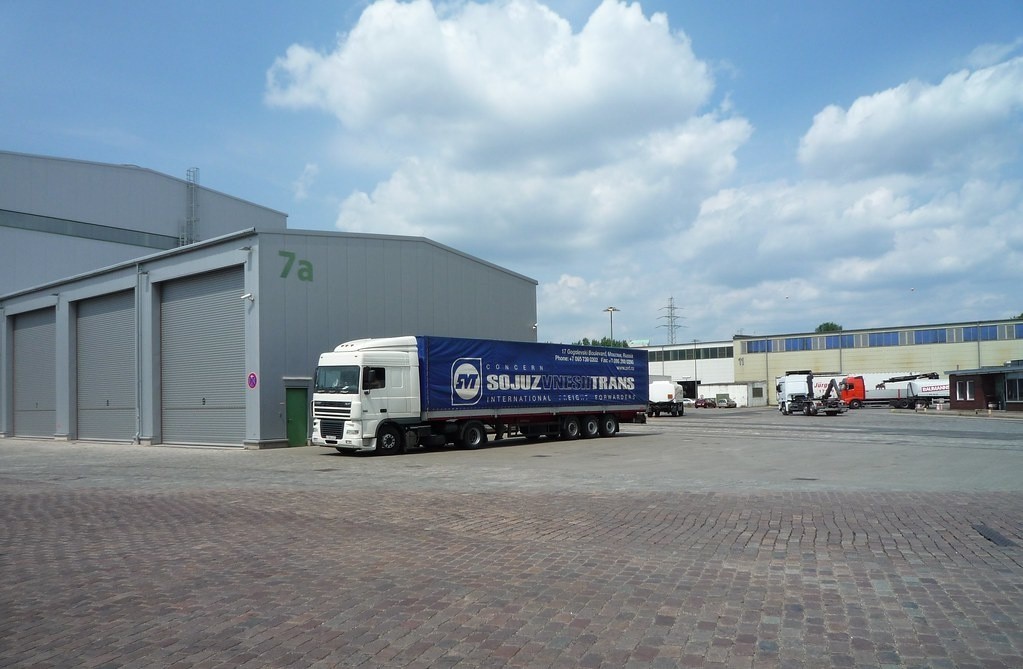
[308,333,651,455]
[833,371,939,410]
[774,374,849,416]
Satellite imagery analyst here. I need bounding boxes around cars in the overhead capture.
[683,398,695,407]
[695,397,716,409]
[717,398,737,408]
